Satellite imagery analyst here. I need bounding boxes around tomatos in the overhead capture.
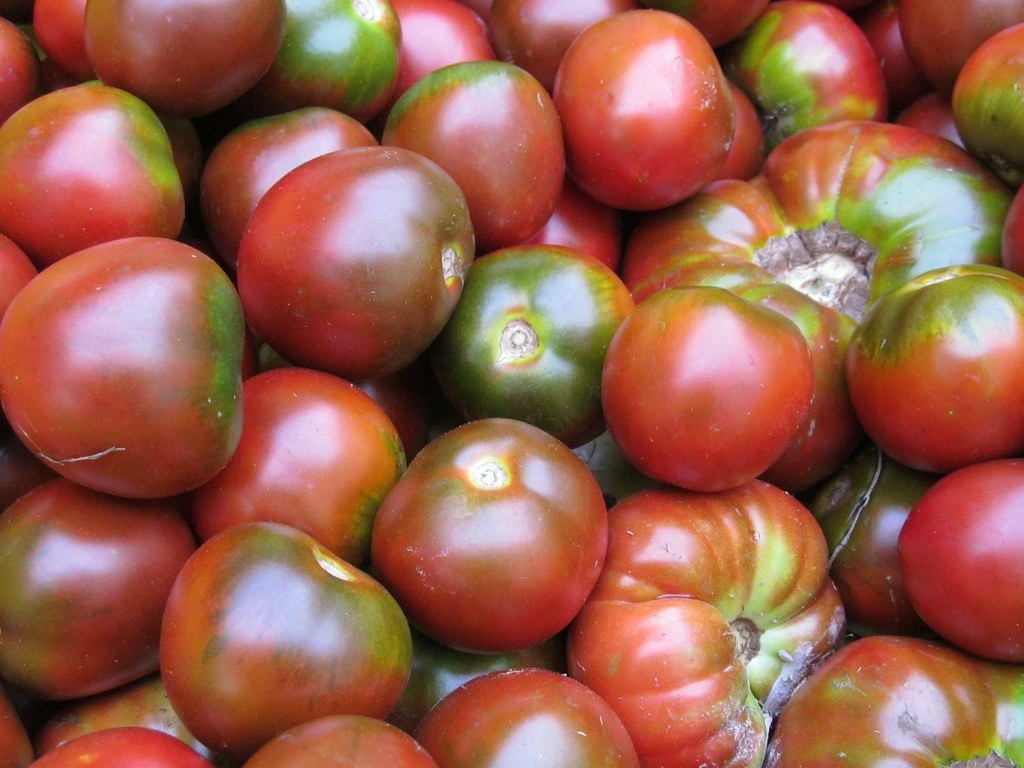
[0,0,1024,768]
